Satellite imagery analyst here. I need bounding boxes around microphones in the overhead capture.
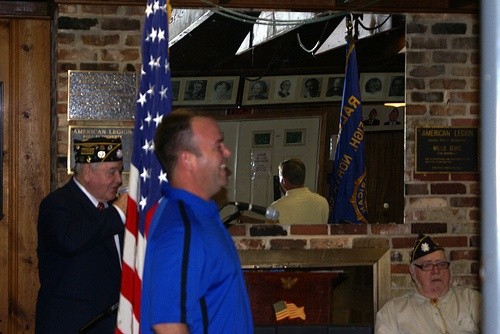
[235,201,279,220]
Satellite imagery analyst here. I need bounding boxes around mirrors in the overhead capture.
[167,0,406,225]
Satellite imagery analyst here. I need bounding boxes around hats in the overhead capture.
[409,233,445,264]
[73,137,124,164]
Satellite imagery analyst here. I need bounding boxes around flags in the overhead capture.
[115,0,173,334]
[327,42,368,223]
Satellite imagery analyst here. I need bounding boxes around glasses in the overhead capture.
[413,262,451,271]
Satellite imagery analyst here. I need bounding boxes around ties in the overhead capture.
[97,202,105,210]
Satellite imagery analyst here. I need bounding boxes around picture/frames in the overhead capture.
[171,72,405,132]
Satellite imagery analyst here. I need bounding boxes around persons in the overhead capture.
[271,158,329,224]
[367,78,381,92]
[326,77,343,96]
[34,137,128,334]
[214,81,229,99]
[392,78,404,96]
[363,108,380,125]
[279,80,291,97]
[385,108,400,125]
[303,78,321,98]
[248,80,267,100]
[184,81,205,100]
[139,109,254,334]
[374,232,485,334]
